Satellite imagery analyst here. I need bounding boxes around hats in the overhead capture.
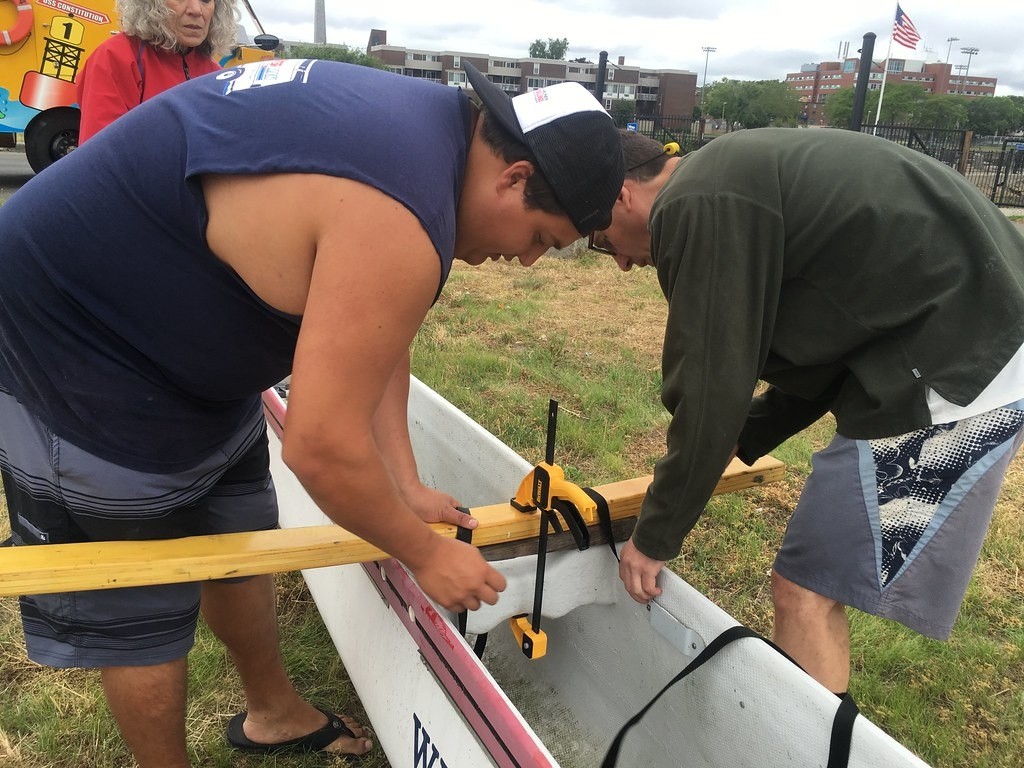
[463,60,625,238]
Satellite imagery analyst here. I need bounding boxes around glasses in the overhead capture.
[588,231,616,256]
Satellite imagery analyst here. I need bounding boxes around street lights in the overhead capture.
[953,47,980,95]
[701,46,717,118]
[945,38,960,65]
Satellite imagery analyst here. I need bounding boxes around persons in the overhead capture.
[586,128,1024,693]
[1,58,627,768]
[78,0,240,147]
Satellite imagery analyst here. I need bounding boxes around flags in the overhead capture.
[892,3,921,51]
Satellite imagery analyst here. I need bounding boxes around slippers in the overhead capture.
[226,706,366,760]
